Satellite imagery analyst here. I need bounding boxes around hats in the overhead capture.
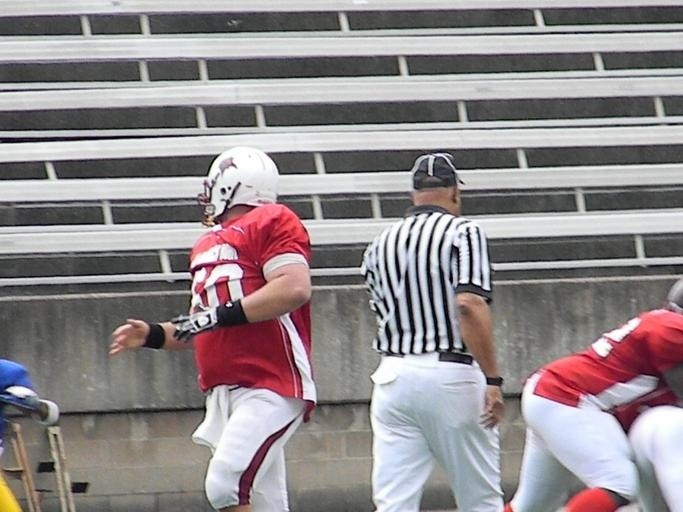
[410,151,466,189]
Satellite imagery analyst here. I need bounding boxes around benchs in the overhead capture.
[0,3,682,304]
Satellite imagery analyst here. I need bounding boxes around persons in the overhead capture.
[626,406,682,511]
[110,146,318,512]
[360,153,504,512]
[505,279,683,512]
[1,360,59,512]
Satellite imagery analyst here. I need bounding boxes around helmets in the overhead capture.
[666,277,683,315]
[197,145,280,227]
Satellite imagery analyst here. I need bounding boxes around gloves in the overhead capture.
[170,303,219,343]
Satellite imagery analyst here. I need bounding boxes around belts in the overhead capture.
[385,351,473,365]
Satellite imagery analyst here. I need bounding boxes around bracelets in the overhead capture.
[486,377,503,385]
[144,323,165,349]
[217,300,249,327]
